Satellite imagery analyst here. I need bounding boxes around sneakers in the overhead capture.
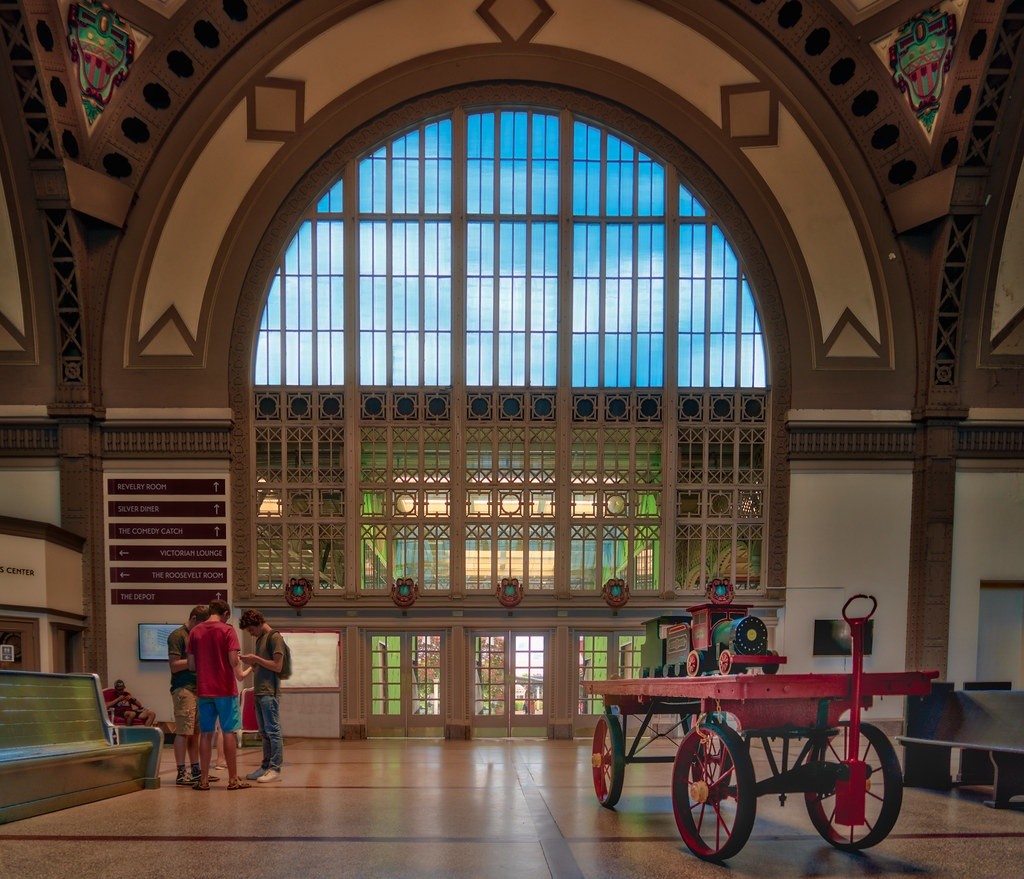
[246,767,266,780]
[257,768,281,783]
[176,770,197,785]
[191,767,220,781]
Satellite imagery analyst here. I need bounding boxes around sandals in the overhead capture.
[227,776,251,789]
[192,779,210,790]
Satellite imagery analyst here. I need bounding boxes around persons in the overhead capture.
[167,605,227,787]
[105,680,156,727]
[237,609,283,784]
[186,600,253,790]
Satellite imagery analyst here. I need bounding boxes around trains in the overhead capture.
[637,578,787,677]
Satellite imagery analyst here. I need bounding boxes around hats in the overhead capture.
[114,679,126,689]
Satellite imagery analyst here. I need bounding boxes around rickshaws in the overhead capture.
[580,593,939,863]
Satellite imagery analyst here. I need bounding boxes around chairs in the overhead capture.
[101,687,146,725]
[211,686,259,749]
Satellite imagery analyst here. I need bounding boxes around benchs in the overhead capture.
[0,671,165,824]
[895,690,1024,809]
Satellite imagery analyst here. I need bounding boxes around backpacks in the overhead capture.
[256,630,292,680]
